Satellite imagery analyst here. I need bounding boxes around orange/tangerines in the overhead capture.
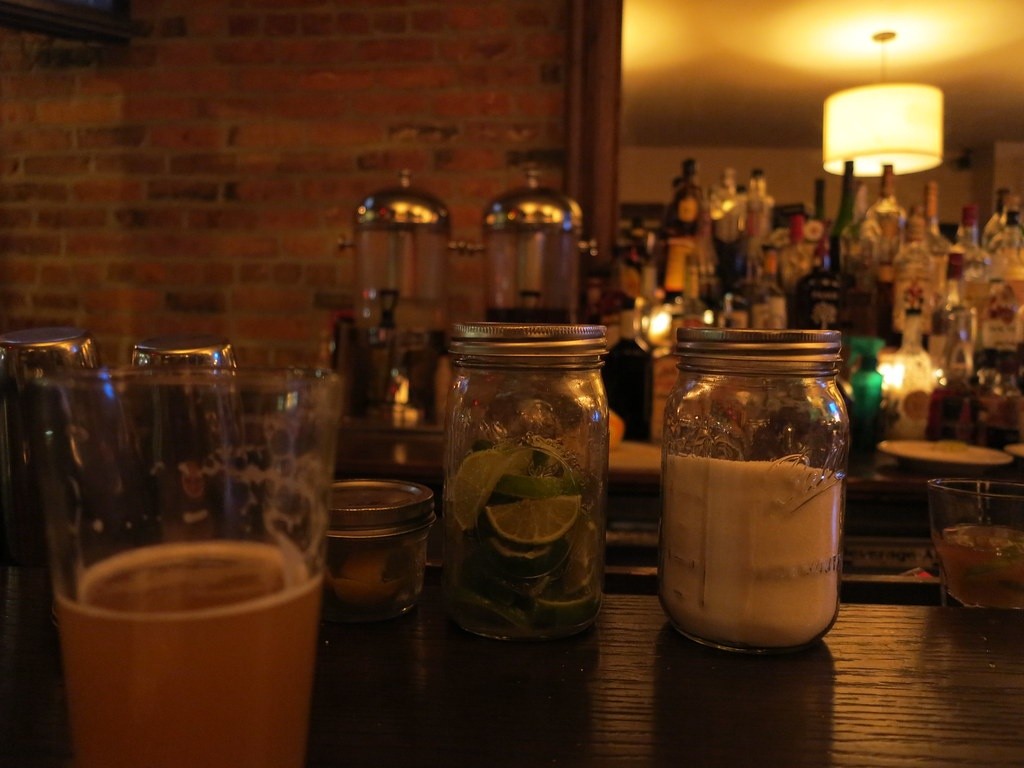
[452,446,596,629]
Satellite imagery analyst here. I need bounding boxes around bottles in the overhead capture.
[627,152,1024,439]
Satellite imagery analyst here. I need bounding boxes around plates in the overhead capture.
[1005,443,1023,457]
[877,440,1014,468]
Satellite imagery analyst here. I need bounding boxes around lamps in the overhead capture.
[821,30,946,175]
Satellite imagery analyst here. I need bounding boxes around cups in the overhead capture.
[24,363,329,767]
[2,325,121,469]
[127,334,250,474]
[926,480,1024,615]
